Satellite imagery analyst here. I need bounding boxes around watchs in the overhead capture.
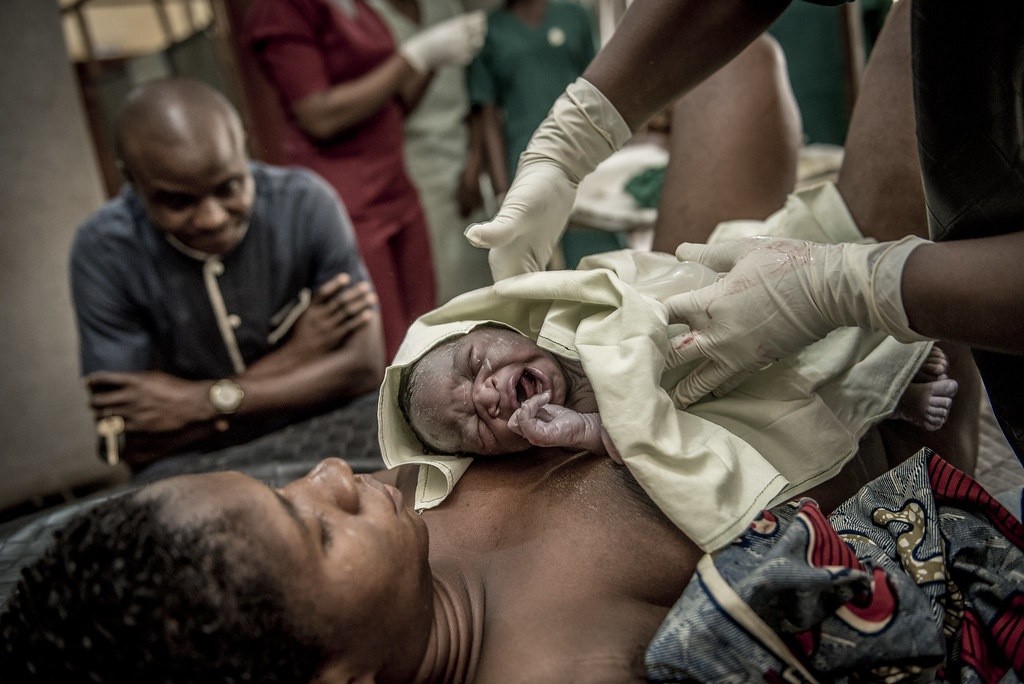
[209,379,243,415]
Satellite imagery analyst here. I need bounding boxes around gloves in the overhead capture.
[397,10,487,78]
[463,77,633,284]
[662,234,939,410]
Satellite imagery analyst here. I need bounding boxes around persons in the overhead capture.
[377,182,957,553]
[0,1,929,683]
[66,76,387,476]
[365,0,497,307]
[474,0,622,269]
[221,0,487,365]
[465,1,1024,468]
